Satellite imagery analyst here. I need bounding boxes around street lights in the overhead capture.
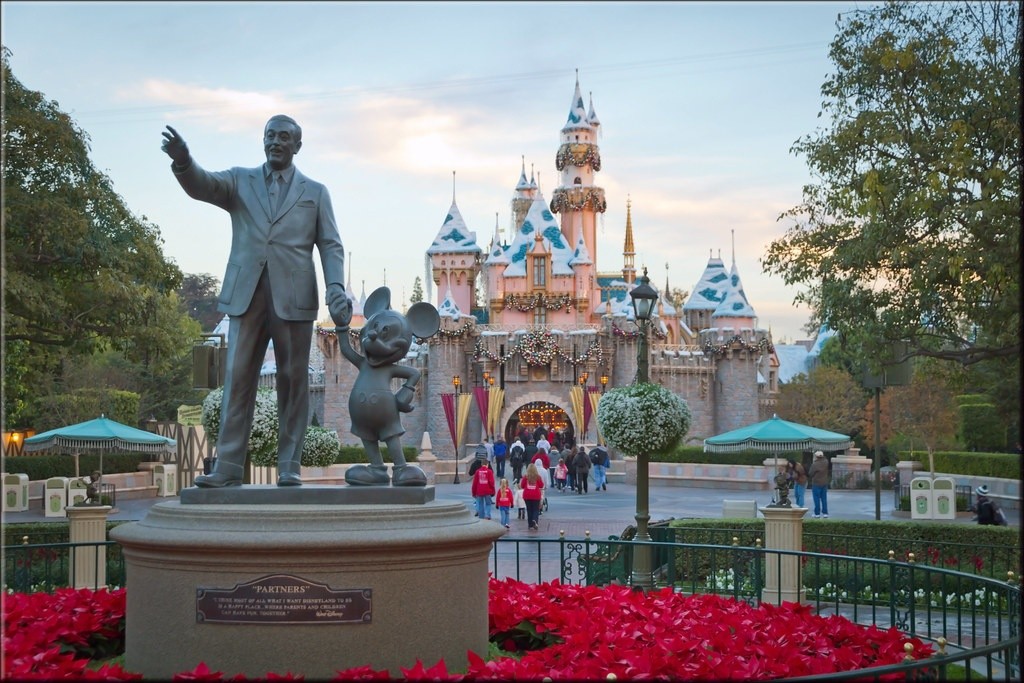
[627,266,658,594]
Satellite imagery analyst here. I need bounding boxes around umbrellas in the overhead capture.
[704,413,851,503]
[24,412,177,506]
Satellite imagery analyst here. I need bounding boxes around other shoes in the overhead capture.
[513,479,518,484]
[596,488,599,491]
[532,520,539,530]
[812,514,820,518]
[505,524,510,528]
[550,482,588,495]
[824,514,829,518]
[602,483,606,491]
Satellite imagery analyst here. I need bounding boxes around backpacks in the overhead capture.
[982,500,1008,526]
[511,448,523,467]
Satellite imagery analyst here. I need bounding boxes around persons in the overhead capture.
[534,459,549,510]
[553,459,568,492]
[161,114,347,488]
[970,484,996,526]
[808,451,829,518]
[788,460,806,508]
[471,459,495,520]
[496,478,514,528]
[468,422,610,521]
[521,463,544,529]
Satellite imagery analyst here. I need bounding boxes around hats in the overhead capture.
[975,484,989,497]
[815,451,824,458]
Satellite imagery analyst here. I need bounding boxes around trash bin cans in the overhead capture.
[68,478,91,506]
[153,464,177,497]
[45,476,69,518]
[2,473,29,512]
[722,500,757,518]
[932,477,956,520]
[910,477,931,519]
